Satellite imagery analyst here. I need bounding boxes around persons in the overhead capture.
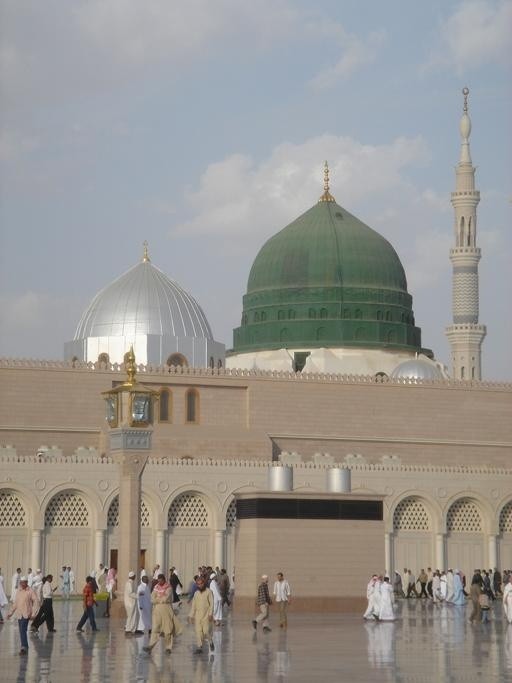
[1,559,238,658]
[272,573,291,628]
[252,575,271,632]
[362,567,512,625]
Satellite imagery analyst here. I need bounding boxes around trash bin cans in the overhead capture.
[92,591,110,618]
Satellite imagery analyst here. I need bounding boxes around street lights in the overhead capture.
[97,343,161,619]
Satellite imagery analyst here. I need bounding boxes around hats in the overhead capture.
[129,572,134,578]
[210,574,216,579]
